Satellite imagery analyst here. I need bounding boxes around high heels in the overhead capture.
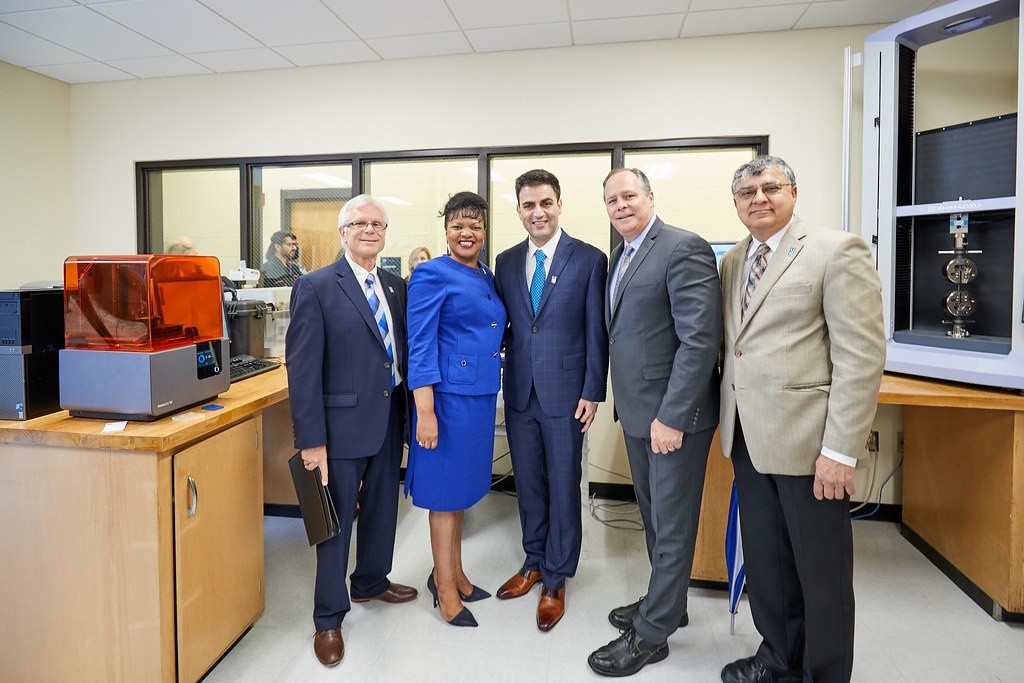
[457,584,491,602]
[427,567,478,627]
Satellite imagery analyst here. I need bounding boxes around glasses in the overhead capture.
[734,183,793,200]
[342,220,388,232]
[292,243,298,247]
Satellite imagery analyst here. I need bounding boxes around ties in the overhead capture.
[741,244,770,324]
[611,246,634,317]
[365,273,395,395]
[530,250,547,317]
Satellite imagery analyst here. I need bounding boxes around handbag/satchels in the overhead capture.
[288,449,340,547]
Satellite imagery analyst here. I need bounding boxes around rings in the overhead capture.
[419,442,424,447]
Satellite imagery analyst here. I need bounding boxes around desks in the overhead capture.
[0,356,302,681]
[687,375,1024,622]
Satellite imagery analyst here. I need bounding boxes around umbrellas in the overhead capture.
[724,480,747,634]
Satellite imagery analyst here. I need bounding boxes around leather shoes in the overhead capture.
[608,593,688,627]
[313,625,344,667]
[721,655,804,683]
[536,582,565,632]
[351,581,418,603]
[588,622,669,677]
[497,565,543,599]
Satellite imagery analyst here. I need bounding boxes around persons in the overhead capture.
[285,194,418,666]
[405,191,506,626]
[717,155,886,683]
[168,244,197,256]
[404,246,431,284]
[262,231,307,288]
[587,167,723,677]
[491,168,606,632]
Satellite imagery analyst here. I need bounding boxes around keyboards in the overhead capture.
[229,353,280,384]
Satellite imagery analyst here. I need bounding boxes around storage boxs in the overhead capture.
[224,287,293,357]
[222,286,267,358]
